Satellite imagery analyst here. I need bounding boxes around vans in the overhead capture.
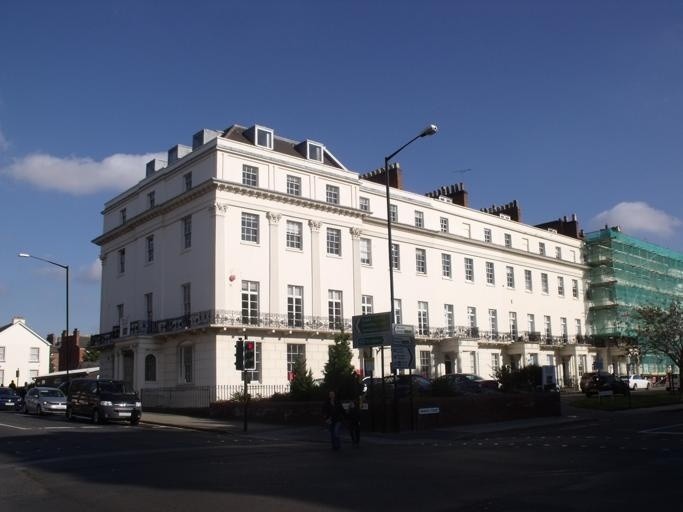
[66,376,143,427]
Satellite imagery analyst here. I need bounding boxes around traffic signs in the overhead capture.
[351,311,394,349]
[391,337,416,369]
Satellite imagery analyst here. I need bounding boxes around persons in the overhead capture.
[9,380,16,390]
[19,387,26,400]
[320,390,347,451]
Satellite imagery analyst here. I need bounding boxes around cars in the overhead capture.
[0,387,25,412]
[23,386,69,418]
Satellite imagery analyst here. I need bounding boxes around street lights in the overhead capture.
[16,251,72,383]
[383,122,439,432]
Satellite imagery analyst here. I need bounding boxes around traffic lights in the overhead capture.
[233,340,237,370]
[244,341,255,370]
